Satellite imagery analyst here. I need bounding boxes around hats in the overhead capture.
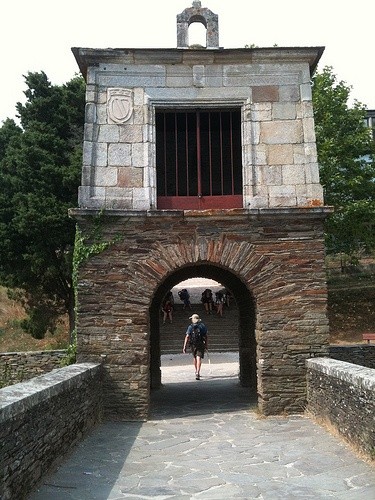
[189,314,200,319]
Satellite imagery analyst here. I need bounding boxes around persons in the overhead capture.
[201,289,214,315]
[216,289,231,317]
[178,289,191,310]
[161,291,174,323]
[183,314,208,380]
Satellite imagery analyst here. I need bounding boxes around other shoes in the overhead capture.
[195,373,200,379]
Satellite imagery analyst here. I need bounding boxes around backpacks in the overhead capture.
[205,290,211,298]
[164,303,171,311]
[191,323,202,343]
